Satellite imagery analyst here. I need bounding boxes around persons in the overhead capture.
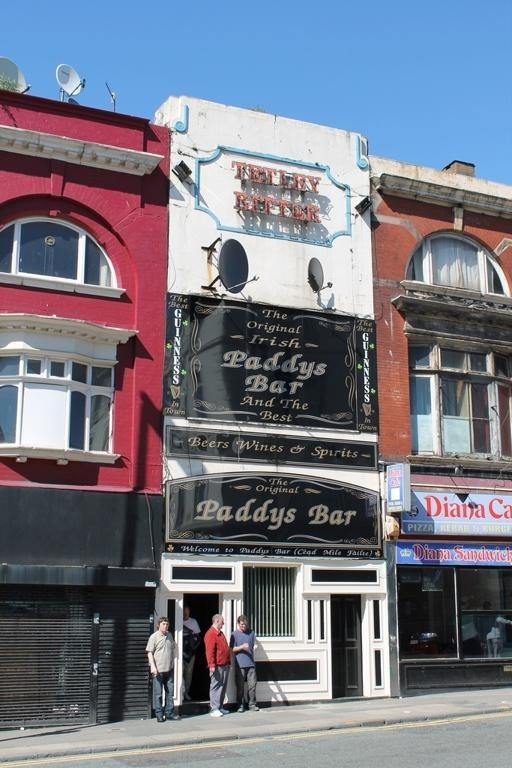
[144,616,182,723]
[472,599,497,656]
[202,613,231,717]
[487,612,512,659]
[181,606,202,700]
[229,614,260,713]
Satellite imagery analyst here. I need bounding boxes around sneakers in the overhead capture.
[247,704,259,711]
[210,708,230,717]
[237,703,244,713]
[157,715,165,722]
[166,713,180,720]
[184,692,191,700]
